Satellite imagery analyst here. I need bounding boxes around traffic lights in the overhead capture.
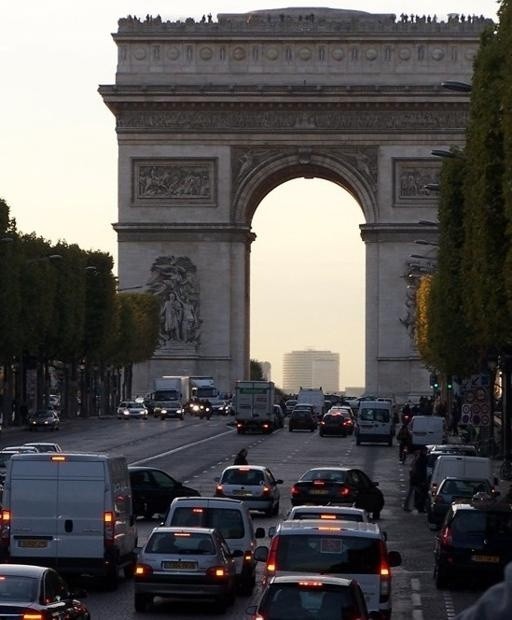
[433,382,438,389]
[447,377,452,390]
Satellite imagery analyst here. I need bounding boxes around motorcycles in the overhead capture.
[401,410,411,423]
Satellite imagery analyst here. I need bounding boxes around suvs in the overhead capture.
[434,499,512,587]
[409,415,448,447]
[161,497,265,597]
[289,404,317,431]
[426,454,495,495]
[254,519,402,620]
[285,400,297,414]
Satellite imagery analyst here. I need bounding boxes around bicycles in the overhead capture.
[398,436,411,463]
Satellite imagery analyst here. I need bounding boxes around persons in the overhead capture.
[234,448,248,464]
[396,391,459,461]
[403,448,430,512]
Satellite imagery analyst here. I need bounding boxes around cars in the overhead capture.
[423,444,478,478]
[213,466,284,517]
[0,564,91,620]
[4,447,38,455]
[320,413,354,436]
[118,401,148,420]
[23,443,62,452]
[350,395,377,408]
[344,396,359,407]
[160,402,184,420]
[0,451,27,491]
[286,505,374,523]
[29,410,59,431]
[128,466,201,519]
[117,401,133,418]
[291,467,384,520]
[274,404,284,427]
[212,400,232,415]
[330,406,354,420]
[246,575,383,620]
[323,395,342,408]
[132,527,244,614]
[42,394,60,409]
[430,476,493,530]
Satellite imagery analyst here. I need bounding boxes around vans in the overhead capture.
[355,400,395,445]
[376,397,396,417]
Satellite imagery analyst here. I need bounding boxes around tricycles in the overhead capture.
[409,398,427,415]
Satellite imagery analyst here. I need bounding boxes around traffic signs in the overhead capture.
[465,391,475,403]
[472,403,480,414]
[462,415,470,424]
[481,414,490,425]
[472,415,480,426]
[463,404,471,415]
[481,403,490,414]
[475,388,487,402]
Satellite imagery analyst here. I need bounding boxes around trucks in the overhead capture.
[234,380,275,433]
[1,452,139,590]
[190,376,220,405]
[153,375,189,414]
[298,385,326,421]
